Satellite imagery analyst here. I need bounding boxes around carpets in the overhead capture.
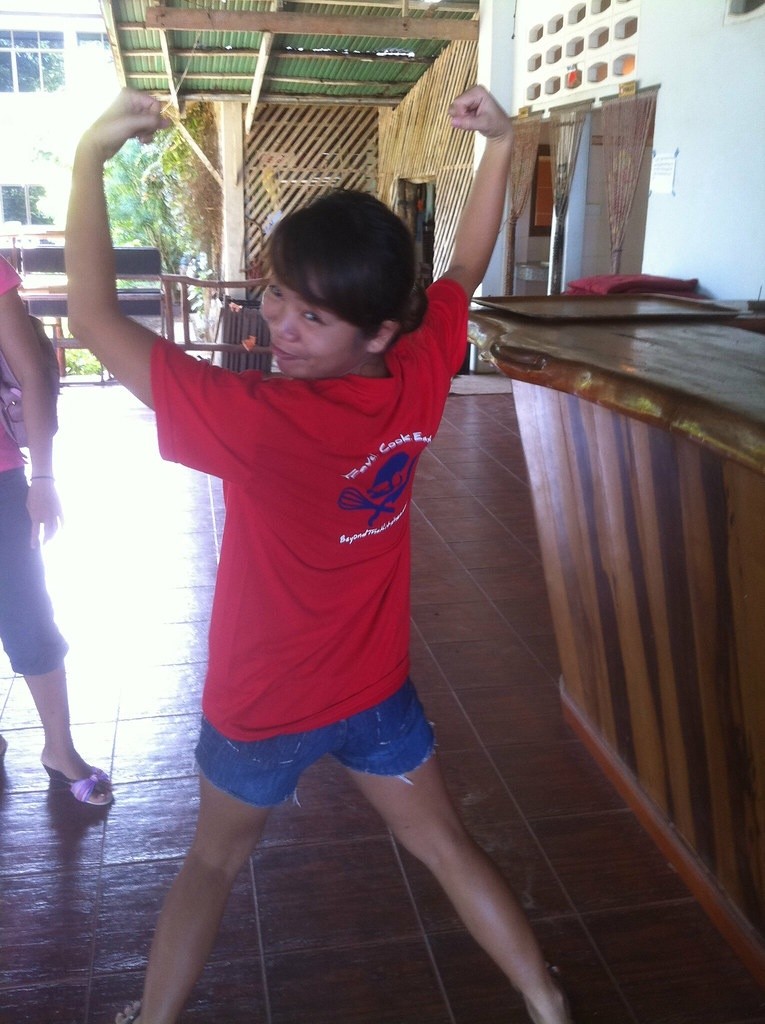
[450,373,513,395]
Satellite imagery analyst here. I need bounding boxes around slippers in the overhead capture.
[41,758,114,805]
[124,1007,143,1024]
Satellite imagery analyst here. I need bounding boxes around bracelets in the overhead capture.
[29,475,55,481]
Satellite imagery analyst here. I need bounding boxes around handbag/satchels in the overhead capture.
[0,314,60,447]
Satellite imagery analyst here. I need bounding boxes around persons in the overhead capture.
[0,251,114,807]
[63,86,569,1023]
